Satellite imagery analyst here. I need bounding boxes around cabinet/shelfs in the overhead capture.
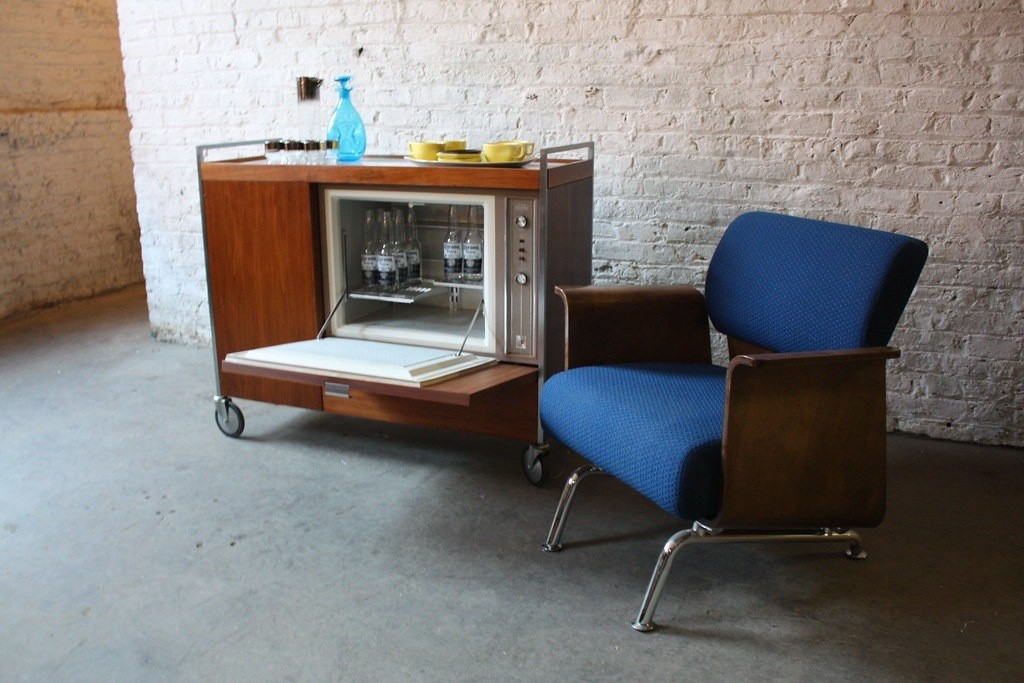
[194,139,594,486]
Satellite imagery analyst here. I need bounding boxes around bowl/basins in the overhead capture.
[437,149,482,162]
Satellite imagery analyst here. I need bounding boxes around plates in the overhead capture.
[404,157,535,166]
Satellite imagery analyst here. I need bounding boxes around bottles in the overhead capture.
[327,75,367,161]
[462,205,483,282]
[443,205,462,281]
[361,208,423,294]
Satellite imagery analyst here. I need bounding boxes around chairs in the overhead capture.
[540,212,930,632]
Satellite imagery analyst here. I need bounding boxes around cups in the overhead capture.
[409,142,446,160]
[482,141,535,161]
[443,140,466,151]
[265,140,337,165]
